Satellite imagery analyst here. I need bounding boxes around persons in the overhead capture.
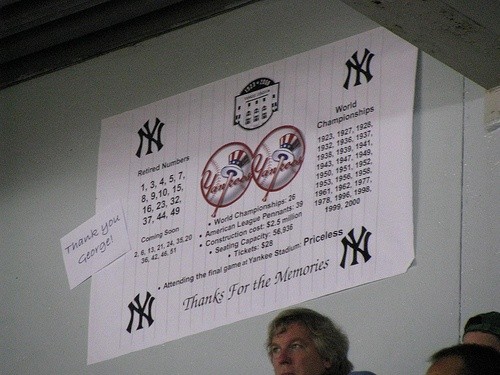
[265,308,376,374]
[424,344,499,375]
[461,311,500,353]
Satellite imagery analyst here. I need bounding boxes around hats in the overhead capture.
[462,311,500,337]
